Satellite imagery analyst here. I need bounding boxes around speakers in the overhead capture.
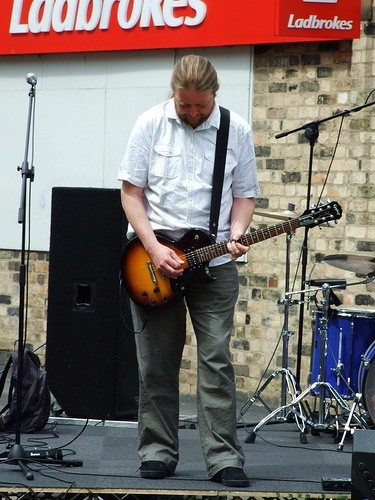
[350,429,375,500]
[44,187,141,423]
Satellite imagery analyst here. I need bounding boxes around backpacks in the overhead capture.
[0,340,50,434]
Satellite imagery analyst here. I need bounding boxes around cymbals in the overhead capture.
[324,254,375,277]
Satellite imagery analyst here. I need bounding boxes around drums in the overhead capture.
[310,306,375,400]
[357,340,375,430]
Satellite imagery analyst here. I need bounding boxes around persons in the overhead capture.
[118,55,260,488]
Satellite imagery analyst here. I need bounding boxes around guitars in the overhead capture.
[121,199,343,311]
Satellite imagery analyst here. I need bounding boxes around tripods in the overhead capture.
[236,218,375,443]
[0,85,83,480]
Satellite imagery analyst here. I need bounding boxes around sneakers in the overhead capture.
[135,460,169,478]
[217,467,250,486]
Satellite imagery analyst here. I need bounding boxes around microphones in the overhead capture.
[25,73,37,86]
